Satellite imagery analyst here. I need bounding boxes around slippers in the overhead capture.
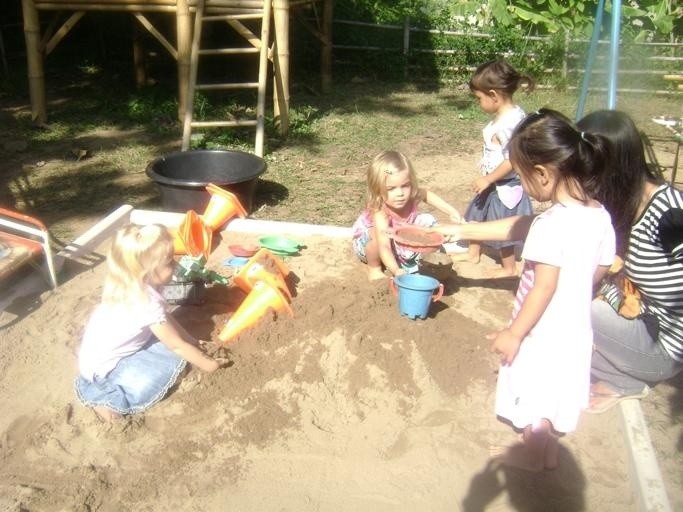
[582,380,651,414]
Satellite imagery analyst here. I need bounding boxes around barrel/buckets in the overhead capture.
[390,272,444,319]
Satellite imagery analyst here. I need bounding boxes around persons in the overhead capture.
[447,60,535,281]
[426,108,683,414]
[72,223,230,426]
[488,106,616,474]
[350,151,469,284]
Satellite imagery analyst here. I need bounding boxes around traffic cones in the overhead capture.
[218,273,292,342]
[171,183,248,261]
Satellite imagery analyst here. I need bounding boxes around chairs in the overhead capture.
[0,209,55,290]
